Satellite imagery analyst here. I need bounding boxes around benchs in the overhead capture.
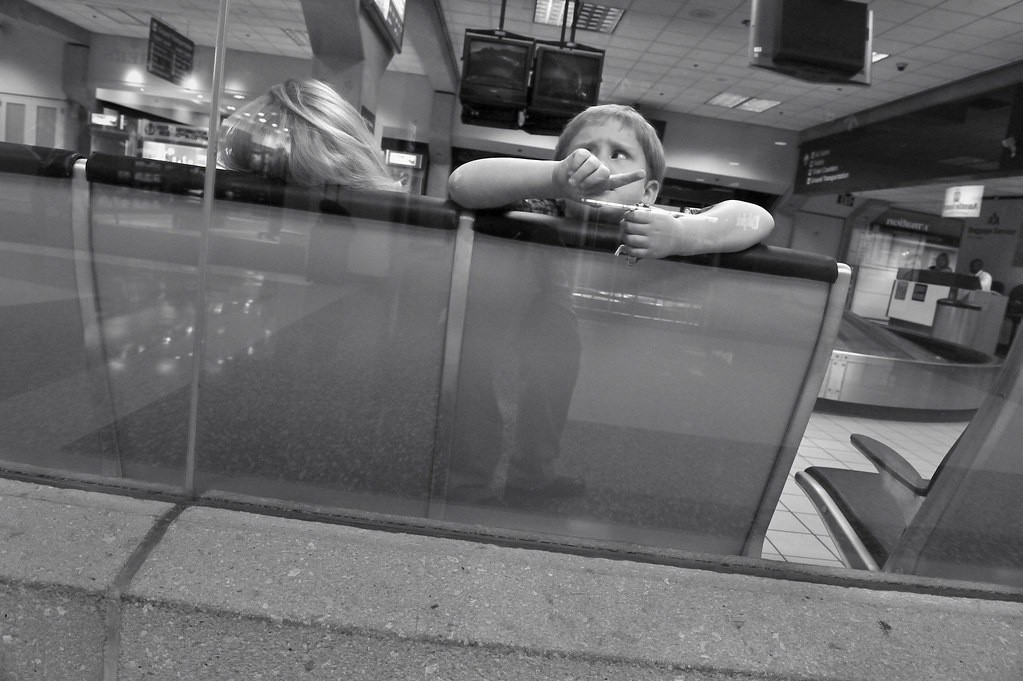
[1,145,1021,593]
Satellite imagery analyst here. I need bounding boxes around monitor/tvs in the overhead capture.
[747,0,873,85]
[458,33,604,117]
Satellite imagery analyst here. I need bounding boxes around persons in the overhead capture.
[220,78,406,195]
[273,211,590,501]
[928,253,952,272]
[970,259,992,290]
[448,103,774,260]
